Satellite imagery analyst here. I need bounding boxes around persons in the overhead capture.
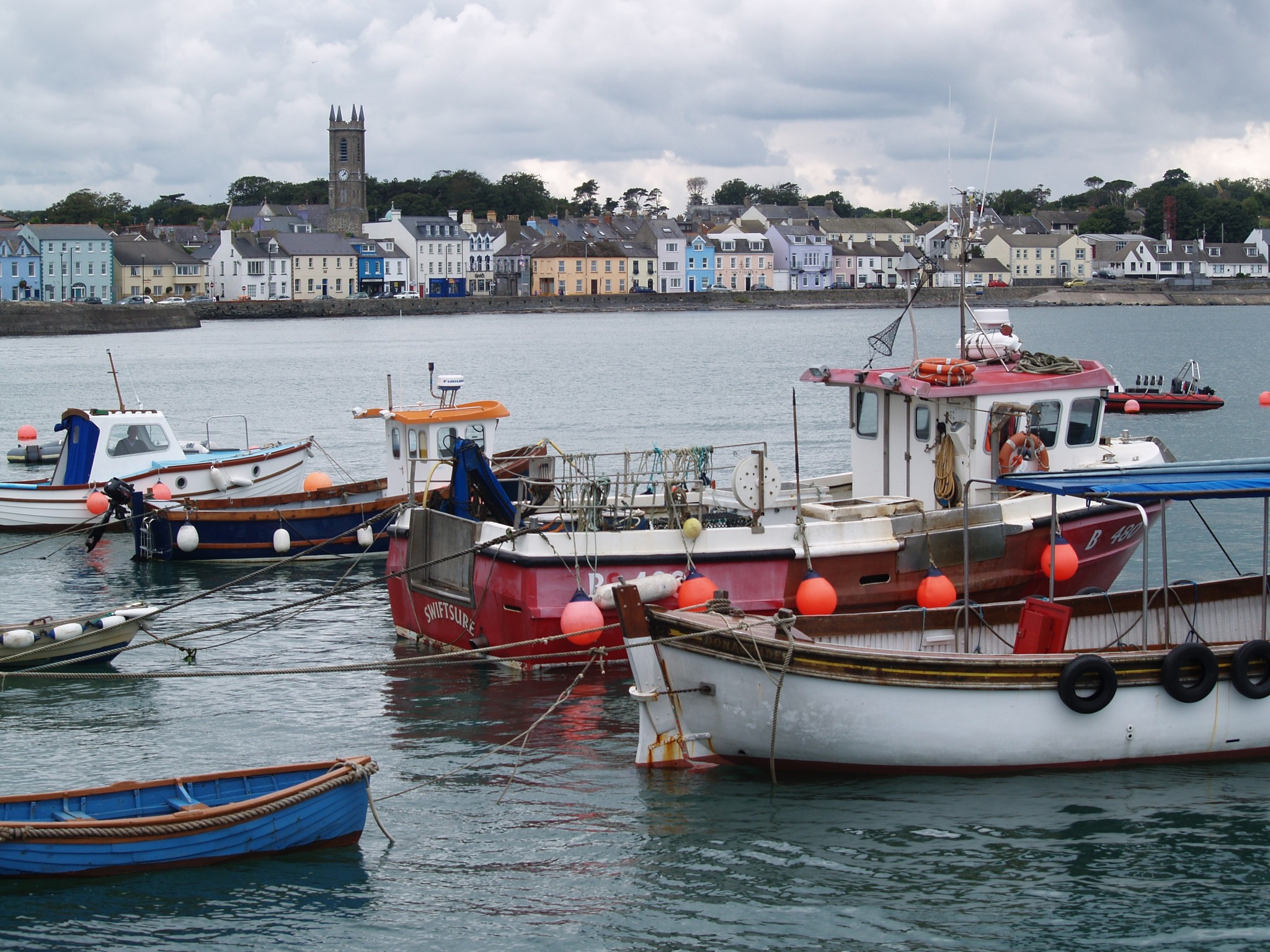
[558,288,563,295]
[212,292,220,302]
[534,287,542,296]
[370,289,374,299]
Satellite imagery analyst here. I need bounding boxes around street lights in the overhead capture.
[70,246,80,297]
[1148,239,1159,285]
[585,242,594,294]
[446,244,454,278]
[141,253,146,304]
[268,246,280,297]
[58,251,65,303]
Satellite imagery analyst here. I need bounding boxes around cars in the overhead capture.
[136,295,154,305]
[229,295,251,301]
[157,297,185,304]
[629,283,774,294]
[265,290,420,301]
[116,296,146,305]
[0,295,40,302]
[1063,268,1116,288]
[964,279,1009,288]
[184,295,212,303]
[824,280,918,290]
[63,296,103,305]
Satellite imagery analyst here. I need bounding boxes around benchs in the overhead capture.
[53,811,96,823]
[167,796,210,811]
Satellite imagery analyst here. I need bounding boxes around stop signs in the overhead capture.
[241,286,246,291]
[210,282,214,288]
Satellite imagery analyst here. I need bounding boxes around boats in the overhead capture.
[608,455,1270,774]
[385,87,1168,674]
[0,599,149,669]
[1051,359,1224,414]
[84,359,557,562]
[6,439,66,464]
[0,755,372,881]
[0,348,317,530]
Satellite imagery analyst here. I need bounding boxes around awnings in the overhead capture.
[633,269,639,274]
[648,269,653,274]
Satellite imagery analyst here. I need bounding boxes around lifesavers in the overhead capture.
[1168,579,1193,586]
[949,599,978,607]
[1021,594,1047,601]
[1172,378,1181,393]
[1076,587,1105,596]
[1000,432,1050,497]
[912,357,977,386]
[896,604,922,611]
[1162,642,1219,704]
[1056,654,1118,716]
[1241,572,1259,577]
[1229,639,1270,700]
